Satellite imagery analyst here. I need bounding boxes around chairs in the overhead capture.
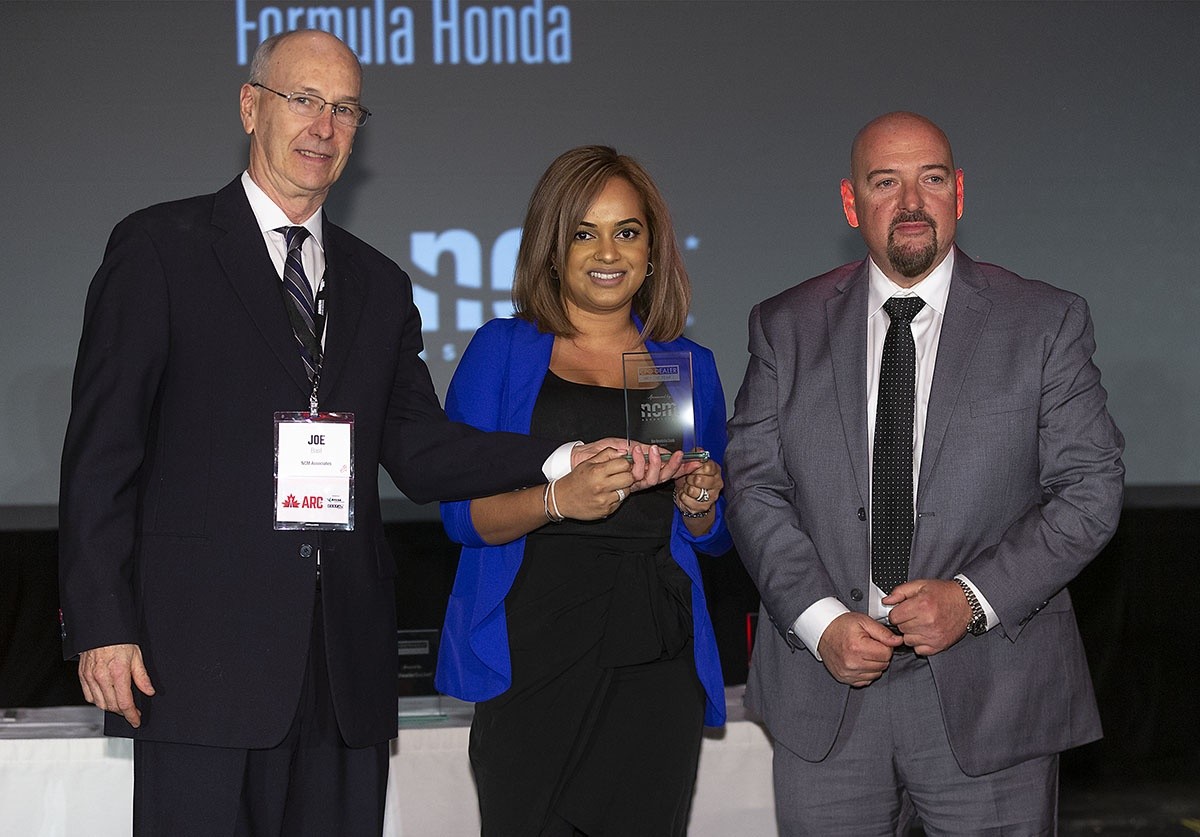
[0,504,1200,780]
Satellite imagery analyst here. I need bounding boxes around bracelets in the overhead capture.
[672,487,711,519]
[543,478,564,523]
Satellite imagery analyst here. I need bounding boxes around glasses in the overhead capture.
[249,83,371,131]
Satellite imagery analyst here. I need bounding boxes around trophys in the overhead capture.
[621,350,710,464]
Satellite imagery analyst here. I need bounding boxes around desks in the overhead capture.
[0,682,781,837]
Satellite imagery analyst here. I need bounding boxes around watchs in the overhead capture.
[954,577,987,637]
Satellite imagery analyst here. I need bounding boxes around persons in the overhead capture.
[723,112,1125,837]
[58,30,701,837]
[434,144,732,837]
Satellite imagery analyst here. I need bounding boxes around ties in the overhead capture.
[273,228,321,398]
[872,296,925,595]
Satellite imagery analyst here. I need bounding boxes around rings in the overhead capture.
[616,489,625,502]
[697,489,710,503]
